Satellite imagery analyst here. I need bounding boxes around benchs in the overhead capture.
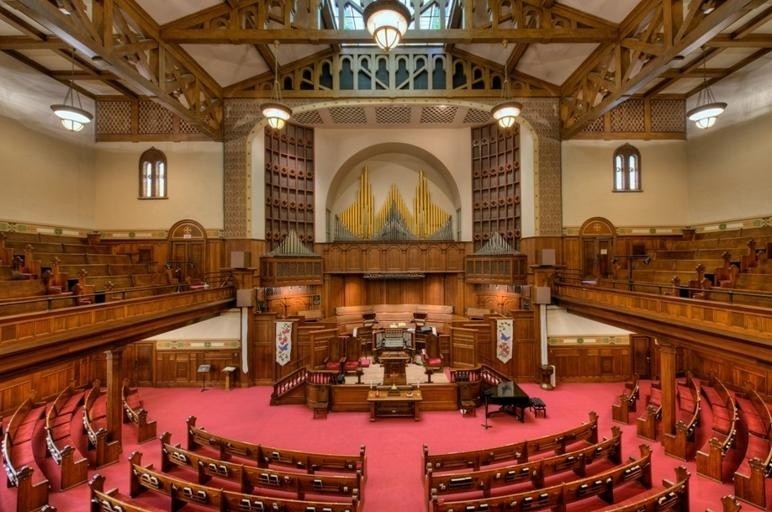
[2,230,212,317]
[563,225,772,314]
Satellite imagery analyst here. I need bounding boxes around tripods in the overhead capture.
[481,396,492,429]
[200,373,207,392]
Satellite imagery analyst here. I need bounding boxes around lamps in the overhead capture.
[258,39,293,131]
[683,45,727,133]
[488,39,521,129]
[47,59,92,131]
[357,0,412,49]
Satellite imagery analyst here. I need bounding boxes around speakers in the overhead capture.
[531,287,551,304]
[536,249,555,265]
[237,289,257,307]
[231,251,249,267]
